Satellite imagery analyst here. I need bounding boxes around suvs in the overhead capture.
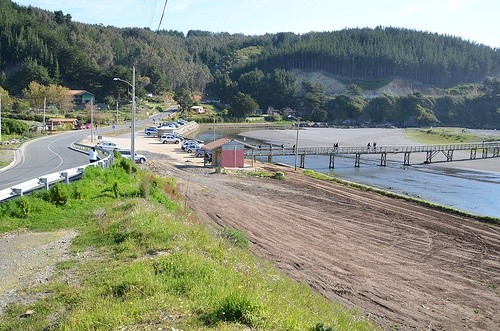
[113,148,147,164]
[185,145,201,154]
[144,122,184,141]
[195,148,204,158]
[161,135,180,144]
[181,140,201,151]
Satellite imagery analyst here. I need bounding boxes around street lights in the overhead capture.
[288,114,300,171]
[113,65,137,164]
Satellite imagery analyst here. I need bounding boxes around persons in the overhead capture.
[333,142,338,150]
[293,144,295,153]
[281,144,284,150]
[373,142,376,150]
[269,144,273,151]
[88,147,97,163]
[367,143,371,150]
[259,143,261,152]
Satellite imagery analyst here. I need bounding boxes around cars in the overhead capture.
[75,122,91,130]
[96,142,120,151]
